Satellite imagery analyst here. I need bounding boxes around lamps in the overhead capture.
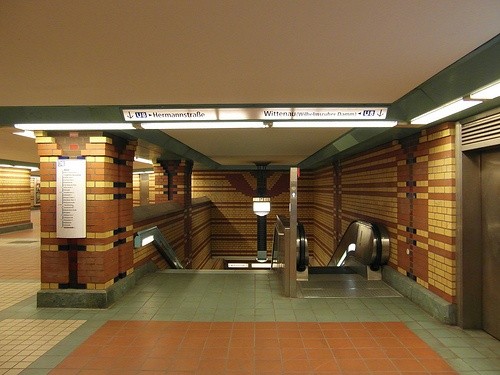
[253,192,271,217]
